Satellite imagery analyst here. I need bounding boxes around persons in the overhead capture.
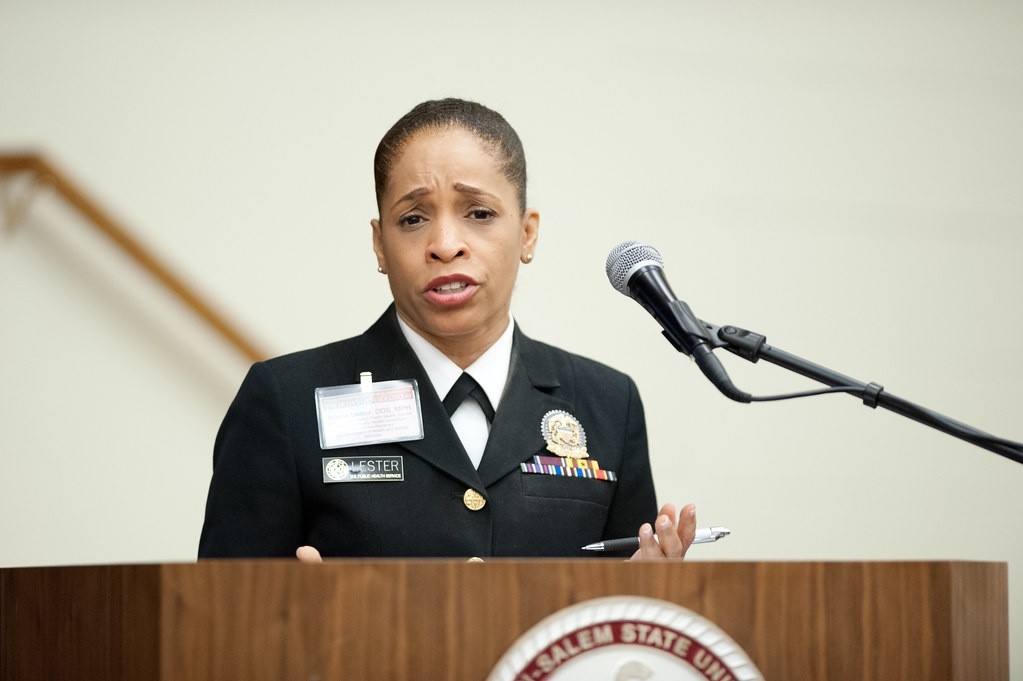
[198,99,696,564]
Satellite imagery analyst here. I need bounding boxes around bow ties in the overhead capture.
[439,373,496,426]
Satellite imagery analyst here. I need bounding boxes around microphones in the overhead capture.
[604,240,752,404]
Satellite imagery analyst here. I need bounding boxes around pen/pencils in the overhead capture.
[581,527,730,551]
